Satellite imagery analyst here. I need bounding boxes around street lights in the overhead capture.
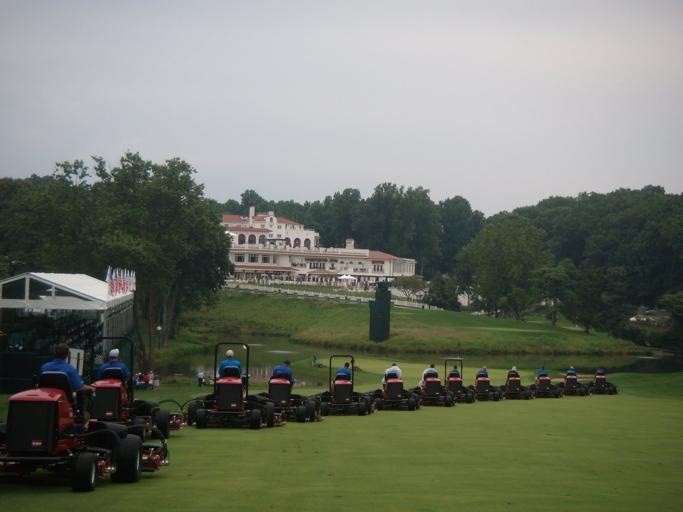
[156,324,162,376]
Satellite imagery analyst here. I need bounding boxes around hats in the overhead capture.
[225,349,235,358]
[108,346,120,359]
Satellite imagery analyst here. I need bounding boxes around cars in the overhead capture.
[628,311,672,327]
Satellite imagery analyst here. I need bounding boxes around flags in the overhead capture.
[105,267,137,297]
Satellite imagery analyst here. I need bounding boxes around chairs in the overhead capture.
[37,366,605,405]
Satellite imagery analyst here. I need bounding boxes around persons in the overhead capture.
[95,346,131,383]
[538,366,549,376]
[335,362,352,380]
[132,368,161,392]
[475,366,489,380]
[566,366,576,375]
[273,359,295,383]
[507,366,519,377]
[382,363,403,383]
[41,342,96,397]
[217,348,243,376]
[446,364,460,380]
[197,370,205,386]
[596,367,606,374]
[419,362,439,386]
